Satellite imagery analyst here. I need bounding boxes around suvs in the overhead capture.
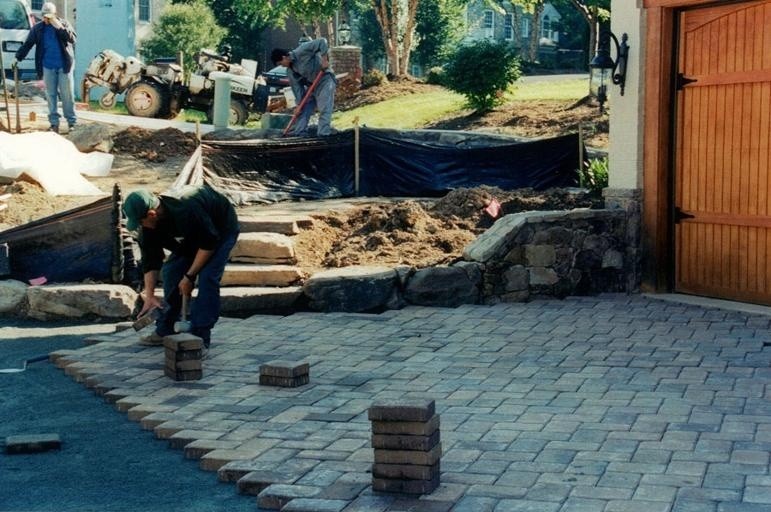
[124,45,254,126]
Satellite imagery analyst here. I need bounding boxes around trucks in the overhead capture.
[0,0,40,81]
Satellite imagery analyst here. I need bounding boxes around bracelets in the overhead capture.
[183,273,194,282]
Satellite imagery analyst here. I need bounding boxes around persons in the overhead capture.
[269,36,337,140]
[11,1,77,136]
[120,186,241,350]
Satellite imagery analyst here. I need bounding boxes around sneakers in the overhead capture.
[47,125,75,132]
[137,329,178,347]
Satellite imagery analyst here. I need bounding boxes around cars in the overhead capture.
[257,64,291,106]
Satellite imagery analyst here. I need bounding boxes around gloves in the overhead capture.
[10,58,19,72]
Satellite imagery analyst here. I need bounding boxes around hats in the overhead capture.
[121,189,157,233]
[42,2,57,19]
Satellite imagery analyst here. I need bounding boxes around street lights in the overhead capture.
[338,20,350,45]
